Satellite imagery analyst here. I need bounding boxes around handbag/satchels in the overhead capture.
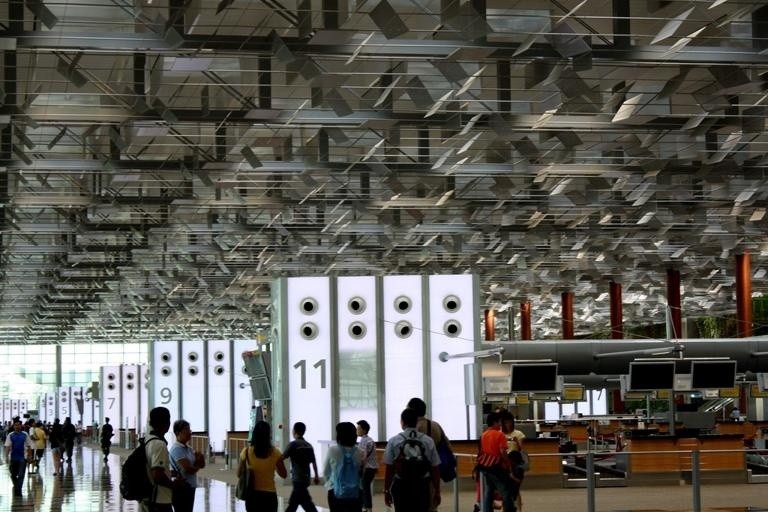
[235,469,257,502]
[511,450,532,472]
[58,445,65,453]
[492,469,520,500]
[171,476,192,508]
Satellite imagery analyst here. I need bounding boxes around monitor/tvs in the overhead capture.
[691,361,737,389]
[628,362,677,392]
[249,376,273,401]
[241,350,267,377]
[510,363,558,394]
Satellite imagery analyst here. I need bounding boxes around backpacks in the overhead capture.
[121,435,167,501]
[389,434,435,503]
[334,446,360,499]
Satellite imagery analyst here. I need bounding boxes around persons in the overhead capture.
[383,397,525,511]
[99,417,116,460]
[133,407,205,511]
[0,417,76,477]
[236,419,378,511]
[4,421,31,497]
